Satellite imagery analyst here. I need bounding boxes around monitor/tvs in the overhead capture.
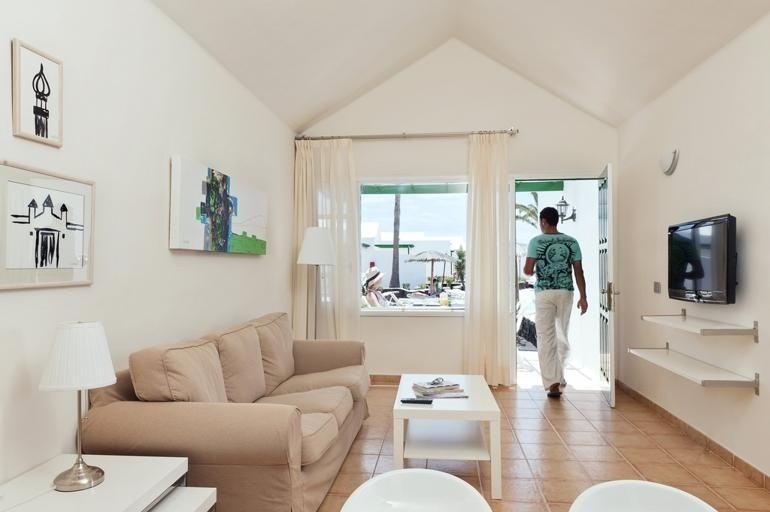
[668,214,735,304]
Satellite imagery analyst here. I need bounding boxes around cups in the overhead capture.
[440,294,447,304]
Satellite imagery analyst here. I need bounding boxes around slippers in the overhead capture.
[547,391,563,397]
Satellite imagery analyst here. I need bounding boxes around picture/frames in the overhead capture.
[11,36,64,150]
[0,158,95,293]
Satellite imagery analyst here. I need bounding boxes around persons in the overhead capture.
[669,227,705,297]
[522,205,591,401]
[435,279,443,294]
[361,266,396,308]
[429,278,437,294]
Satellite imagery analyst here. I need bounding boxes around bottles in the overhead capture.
[448,291,451,305]
[402,280,410,290]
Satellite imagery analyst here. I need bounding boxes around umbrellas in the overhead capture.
[404,250,458,295]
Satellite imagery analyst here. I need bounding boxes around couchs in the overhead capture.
[74,310,374,512]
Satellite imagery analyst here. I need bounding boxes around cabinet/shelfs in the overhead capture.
[0,449,221,512]
[624,307,763,397]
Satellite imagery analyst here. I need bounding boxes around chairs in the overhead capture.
[565,477,721,512]
[360,284,528,349]
[336,468,496,512]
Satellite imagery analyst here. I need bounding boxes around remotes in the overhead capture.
[401,397,433,403]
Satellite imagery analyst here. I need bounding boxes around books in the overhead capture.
[411,380,469,400]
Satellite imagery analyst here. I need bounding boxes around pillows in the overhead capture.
[245,310,297,400]
[125,337,228,415]
[208,323,267,405]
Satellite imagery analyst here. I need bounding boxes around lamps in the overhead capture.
[292,224,341,339]
[33,318,120,495]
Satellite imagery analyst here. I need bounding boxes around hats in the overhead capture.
[363,267,383,288]
